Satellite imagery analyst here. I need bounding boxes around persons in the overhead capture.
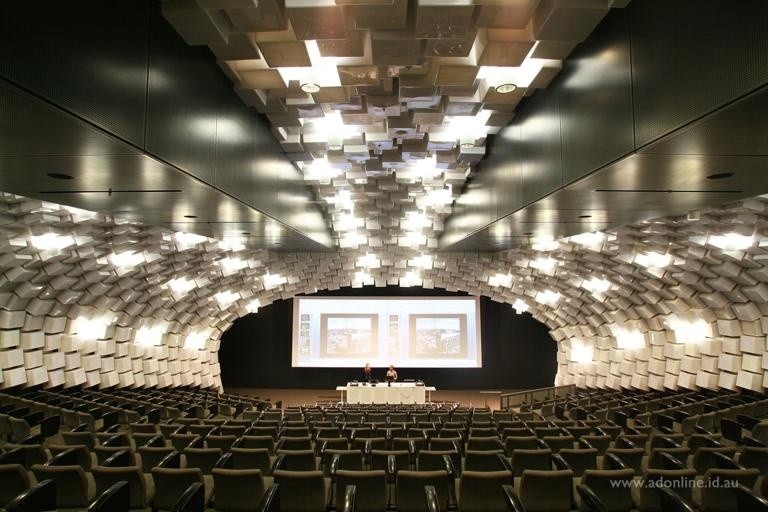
[362,363,378,383]
[385,365,398,382]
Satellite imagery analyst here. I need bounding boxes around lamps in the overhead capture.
[495,83,518,94]
[299,82,322,95]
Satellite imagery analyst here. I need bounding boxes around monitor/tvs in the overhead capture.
[386,376,393,382]
